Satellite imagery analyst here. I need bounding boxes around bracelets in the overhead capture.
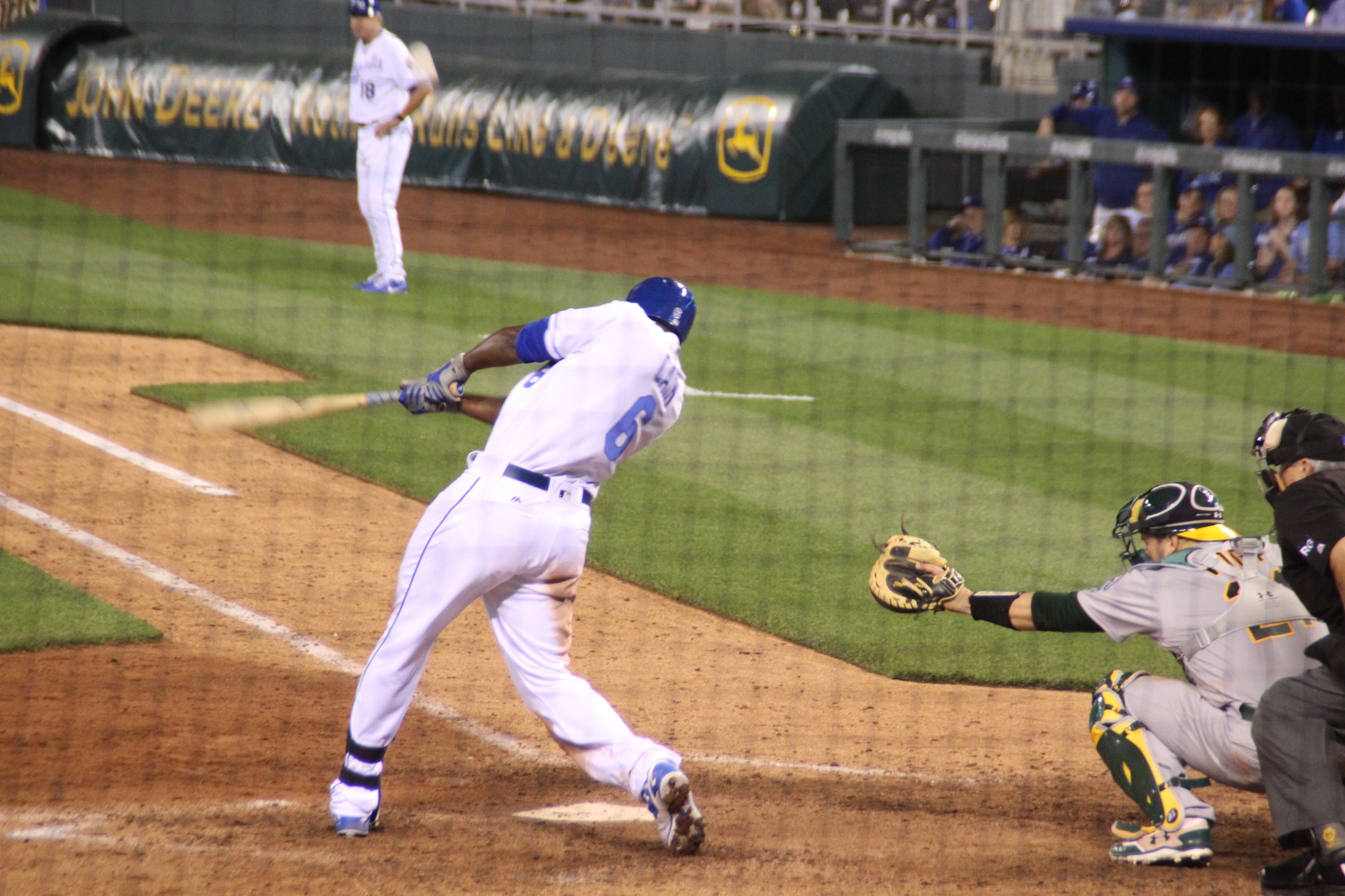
[397,114,404,121]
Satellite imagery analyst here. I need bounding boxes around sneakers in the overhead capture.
[1108,818,1213,868]
[641,762,706,854]
[354,273,408,293]
[1260,846,1345,896]
[335,807,382,837]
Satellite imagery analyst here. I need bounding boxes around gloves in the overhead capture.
[426,352,473,404]
[399,381,459,415]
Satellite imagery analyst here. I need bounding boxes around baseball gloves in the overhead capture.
[868,534,965,615]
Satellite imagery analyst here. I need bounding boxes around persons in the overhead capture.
[330,275,708,855]
[351,0,429,293]
[863,0,1344,305]
[870,407,1345,896]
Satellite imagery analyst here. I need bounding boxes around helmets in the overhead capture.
[1142,481,1244,542]
[626,276,697,344]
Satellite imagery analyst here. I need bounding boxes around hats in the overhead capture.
[1069,78,1099,102]
[1111,74,1141,91]
[958,196,984,211]
[1273,412,1345,464]
[350,0,380,16]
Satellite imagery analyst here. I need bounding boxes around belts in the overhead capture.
[1240,704,1256,720]
[504,464,593,505]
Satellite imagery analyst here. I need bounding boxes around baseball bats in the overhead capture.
[184,379,465,432]
